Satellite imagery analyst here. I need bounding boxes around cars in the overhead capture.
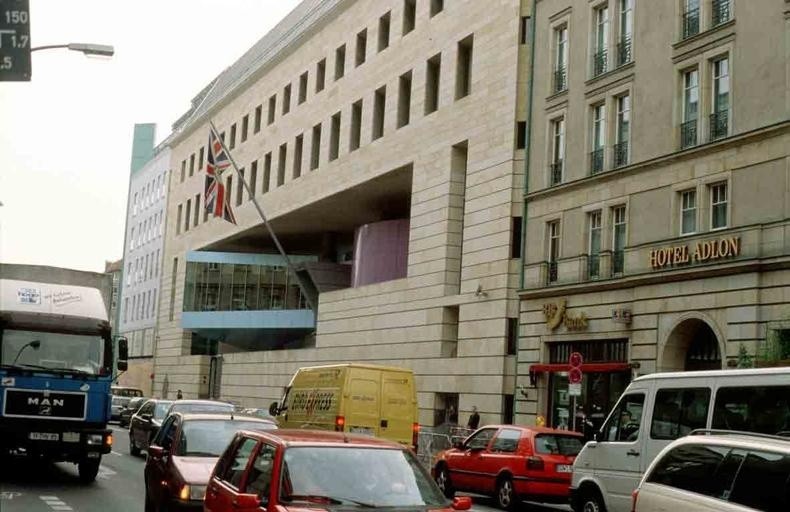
[166,399,233,422]
[129,399,178,456]
[144,411,280,512]
[433,421,586,507]
[202,428,473,512]
[110,387,148,426]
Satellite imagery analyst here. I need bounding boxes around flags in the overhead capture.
[202,125,243,227]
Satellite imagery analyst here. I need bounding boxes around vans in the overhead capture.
[566,365,789,512]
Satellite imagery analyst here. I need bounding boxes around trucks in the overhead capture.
[267,362,419,455]
[0,264,129,487]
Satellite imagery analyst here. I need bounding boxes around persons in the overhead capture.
[465,404,480,437]
[446,405,458,426]
[615,408,637,430]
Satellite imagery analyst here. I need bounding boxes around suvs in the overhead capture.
[628,427,790,512]
